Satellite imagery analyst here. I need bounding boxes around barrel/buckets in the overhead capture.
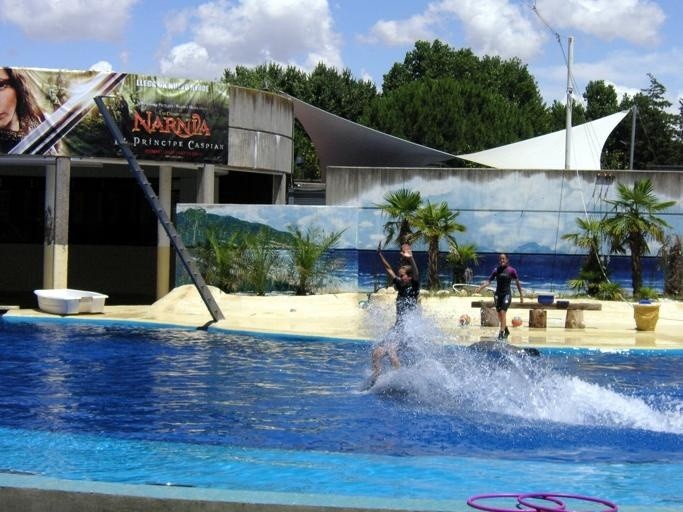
[632,301,661,330]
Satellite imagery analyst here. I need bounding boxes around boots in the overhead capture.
[499,327,509,340]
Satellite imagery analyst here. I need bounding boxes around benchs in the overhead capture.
[472,302,601,328]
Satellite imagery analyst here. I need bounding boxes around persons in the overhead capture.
[0,67,59,157]
[364,240,420,390]
[475,254,523,341]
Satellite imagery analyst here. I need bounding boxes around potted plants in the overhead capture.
[633,287,661,331]
